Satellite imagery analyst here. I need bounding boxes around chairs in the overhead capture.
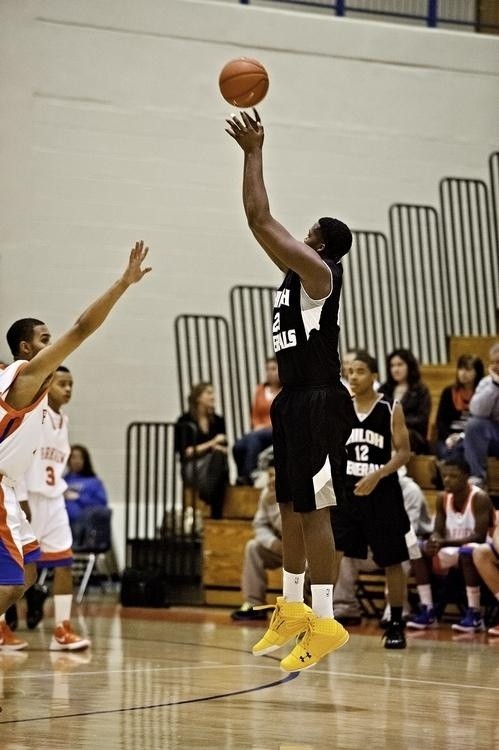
[35,504,113,603]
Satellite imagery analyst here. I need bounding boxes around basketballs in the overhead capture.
[218,57,268,108]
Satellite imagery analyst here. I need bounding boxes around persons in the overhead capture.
[332,465,431,626]
[329,352,408,647]
[233,357,282,487]
[232,459,284,622]
[464,344,499,489]
[226,109,355,673]
[4,584,48,629]
[0,366,91,652]
[175,382,229,520]
[376,350,431,456]
[0,241,152,616]
[341,350,382,397]
[61,445,108,554]
[472,524,499,637]
[407,458,496,634]
[428,354,485,491]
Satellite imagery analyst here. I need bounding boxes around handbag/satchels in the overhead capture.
[121,565,171,608]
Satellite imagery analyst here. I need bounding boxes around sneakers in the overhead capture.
[231,604,267,622]
[279,615,351,672]
[252,597,313,657]
[381,615,406,649]
[334,613,362,626]
[487,624,499,635]
[50,621,90,651]
[406,606,439,629]
[0,620,30,651]
[453,609,488,632]
